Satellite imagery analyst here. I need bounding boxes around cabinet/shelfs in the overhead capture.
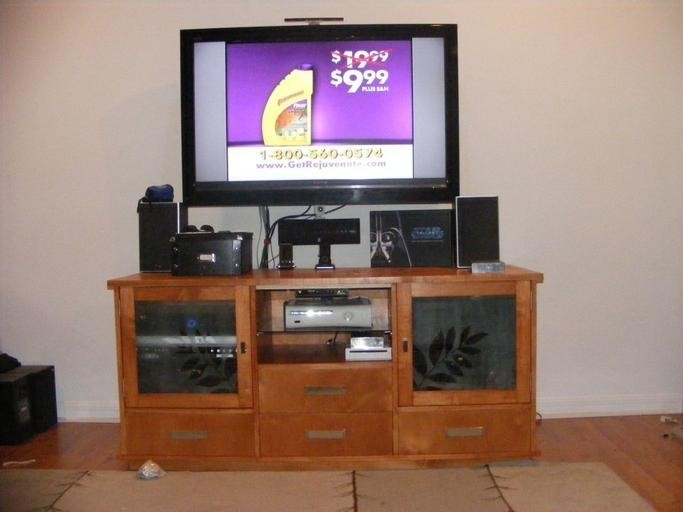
[106,267,543,472]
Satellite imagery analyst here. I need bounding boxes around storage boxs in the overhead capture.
[170,231,253,277]
[0,364,57,445]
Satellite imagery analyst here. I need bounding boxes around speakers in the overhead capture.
[277,218,362,246]
[455,195,499,268]
[137,201,187,274]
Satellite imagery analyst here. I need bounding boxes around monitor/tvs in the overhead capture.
[178,22,460,218]
